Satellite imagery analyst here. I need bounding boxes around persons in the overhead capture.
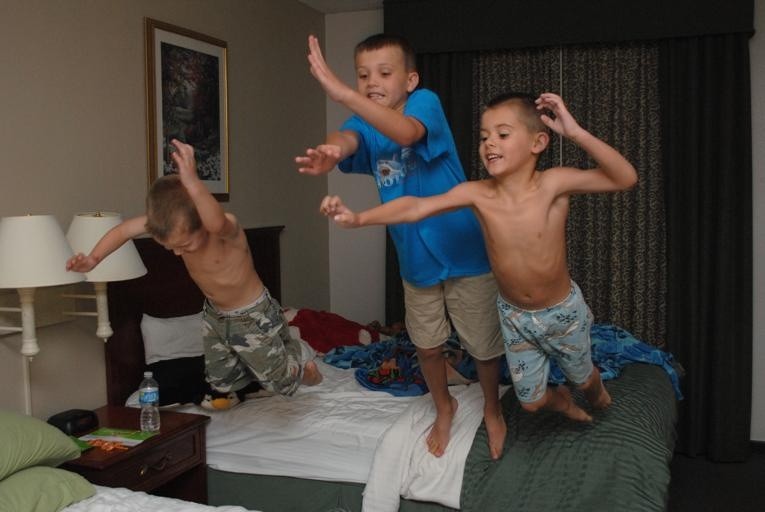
[293,30,509,463]
[61,136,323,398]
[316,91,640,421]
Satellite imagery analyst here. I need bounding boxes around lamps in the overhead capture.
[0,210,148,362]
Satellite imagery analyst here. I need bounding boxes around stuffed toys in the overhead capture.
[194,376,244,411]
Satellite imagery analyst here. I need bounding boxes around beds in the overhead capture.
[60,483,263,512]
[103,225,684,512]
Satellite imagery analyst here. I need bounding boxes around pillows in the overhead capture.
[0,465,96,512]
[0,408,82,482]
[140,310,207,365]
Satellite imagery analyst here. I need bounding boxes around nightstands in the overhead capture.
[57,404,211,504]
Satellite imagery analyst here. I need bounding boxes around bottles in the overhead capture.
[139,372,161,431]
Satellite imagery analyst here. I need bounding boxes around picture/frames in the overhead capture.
[144,15,231,204]
